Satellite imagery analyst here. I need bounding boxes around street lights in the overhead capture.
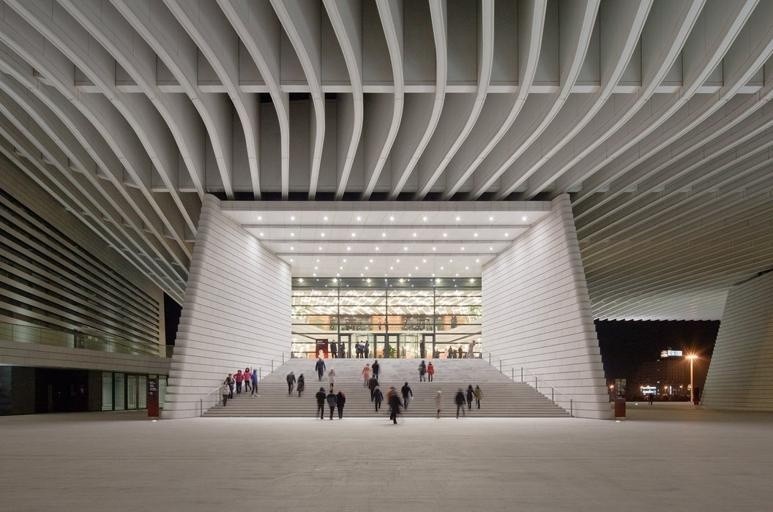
[685,351,697,402]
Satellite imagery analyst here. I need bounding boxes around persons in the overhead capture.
[220,338,483,425]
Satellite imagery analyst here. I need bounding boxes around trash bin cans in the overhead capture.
[148,391,159,417]
[615,395,625,417]
